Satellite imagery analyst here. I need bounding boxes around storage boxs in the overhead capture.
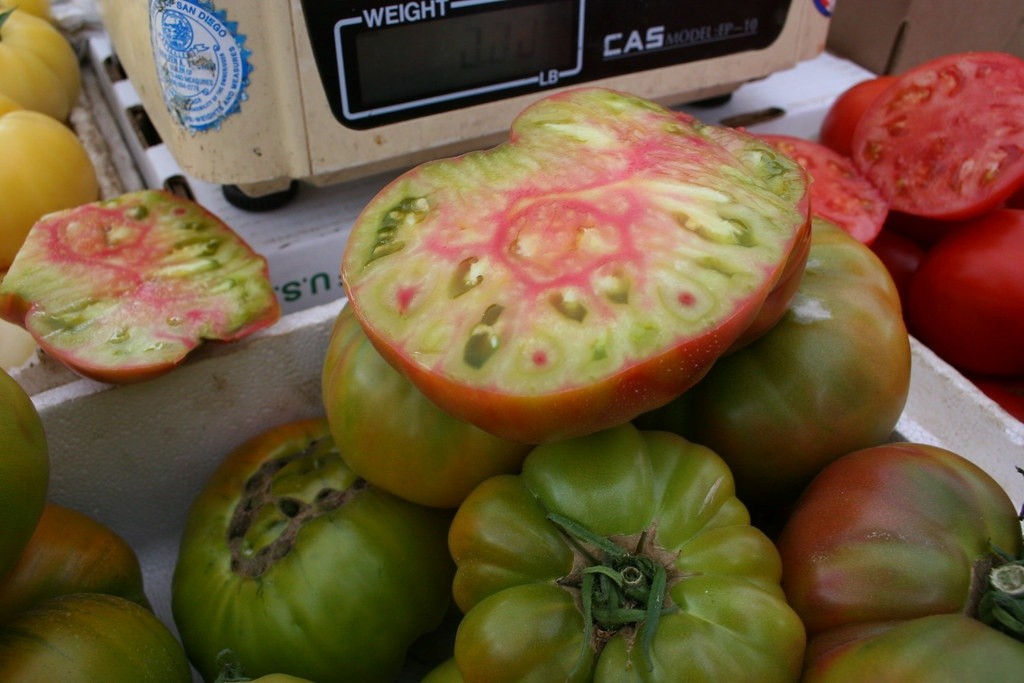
[0,0,1024,683]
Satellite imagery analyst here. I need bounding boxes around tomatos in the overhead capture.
[0,0,1024,683]
